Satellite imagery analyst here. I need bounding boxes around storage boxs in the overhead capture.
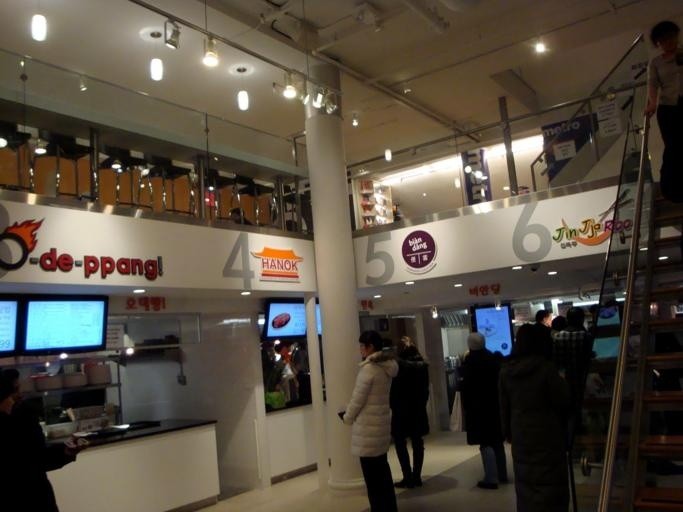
[89,364,112,386]
[30,373,63,388]
[67,405,110,432]
[40,415,76,439]
[63,373,88,387]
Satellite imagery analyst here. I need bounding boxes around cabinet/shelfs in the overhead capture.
[361,179,393,228]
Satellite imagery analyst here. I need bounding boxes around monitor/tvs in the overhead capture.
[20,294,109,356]
[263,297,307,339]
[0,293,22,359]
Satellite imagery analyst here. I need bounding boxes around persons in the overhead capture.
[228,207,253,225]
[0,367,87,512]
[343,329,398,510]
[300,183,313,235]
[261,339,312,412]
[390,342,430,489]
[455,332,511,490]
[502,306,604,510]
[643,19,682,195]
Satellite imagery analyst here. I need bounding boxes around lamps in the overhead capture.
[79,77,86,93]
[202,38,217,68]
[299,90,310,104]
[312,87,324,109]
[325,91,338,114]
[165,29,181,51]
[464,160,490,182]
[282,72,297,100]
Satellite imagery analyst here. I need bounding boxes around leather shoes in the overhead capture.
[476,482,497,489]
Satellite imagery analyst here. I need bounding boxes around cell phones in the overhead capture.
[64,437,90,449]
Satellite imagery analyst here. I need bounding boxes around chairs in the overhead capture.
[0,138,274,227]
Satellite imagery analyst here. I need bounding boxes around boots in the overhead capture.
[413,468,421,486]
[394,474,416,488]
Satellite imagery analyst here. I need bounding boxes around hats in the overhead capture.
[467,333,484,351]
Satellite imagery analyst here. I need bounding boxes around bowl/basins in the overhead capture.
[83,366,111,385]
[31,375,62,392]
[61,373,86,388]
[77,417,109,431]
[42,421,77,439]
